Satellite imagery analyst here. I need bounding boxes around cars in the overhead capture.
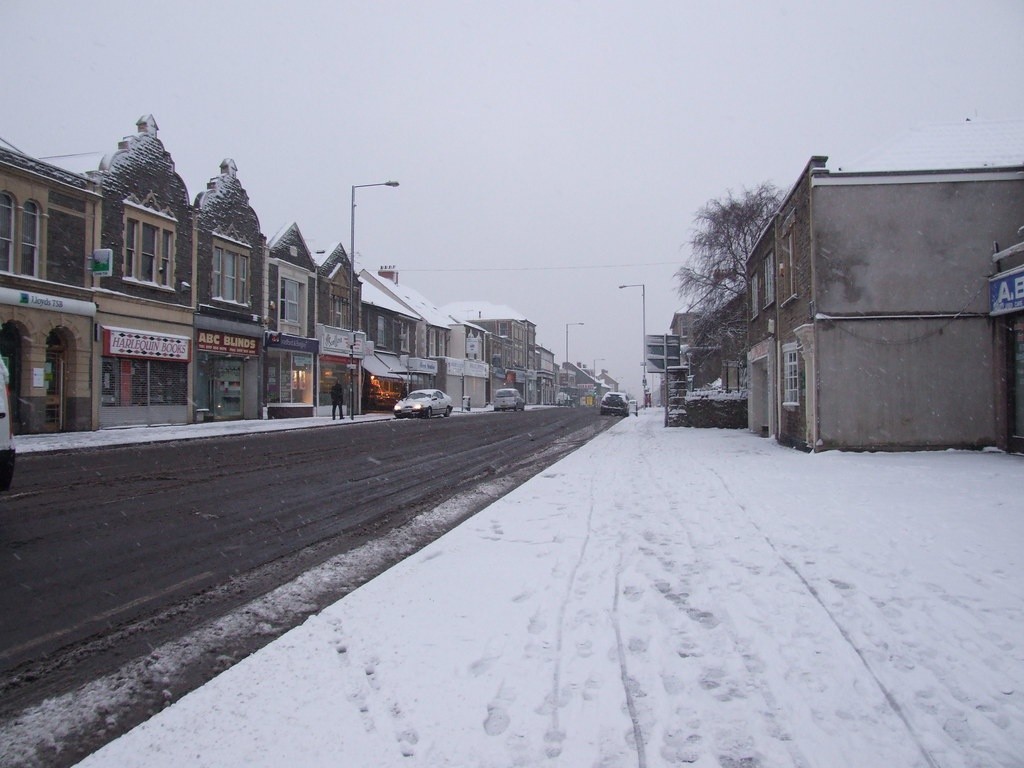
[393,389,453,420]
[494,389,526,413]
[600,392,631,417]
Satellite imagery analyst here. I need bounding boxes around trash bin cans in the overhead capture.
[463,395,471,411]
[629,400,638,417]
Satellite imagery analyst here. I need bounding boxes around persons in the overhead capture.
[331,379,344,420]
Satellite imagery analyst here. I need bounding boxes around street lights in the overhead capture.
[349,180,400,422]
[593,358,606,383]
[619,284,647,389]
[565,323,585,384]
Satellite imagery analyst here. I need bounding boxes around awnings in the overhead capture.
[360,352,422,381]
